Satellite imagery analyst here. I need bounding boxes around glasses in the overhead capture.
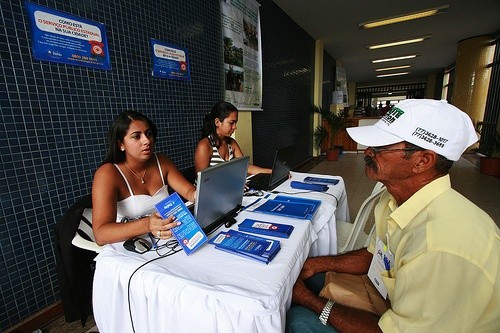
[372,147,444,163]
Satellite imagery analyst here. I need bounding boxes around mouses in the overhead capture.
[123,236,152,254]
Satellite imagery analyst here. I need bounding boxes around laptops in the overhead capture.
[186,156,250,235]
[245,143,297,191]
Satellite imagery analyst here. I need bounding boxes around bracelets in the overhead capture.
[318,300,333,325]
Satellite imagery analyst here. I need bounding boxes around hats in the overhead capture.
[345,98,481,162]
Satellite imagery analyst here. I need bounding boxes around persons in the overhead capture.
[194,101,292,190]
[92,109,197,247]
[354,101,366,118]
[285,99,500,333]
[381,100,391,113]
[379,101,382,109]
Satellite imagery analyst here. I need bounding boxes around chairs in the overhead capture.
[335,181,388,256]
[65,202,102,253]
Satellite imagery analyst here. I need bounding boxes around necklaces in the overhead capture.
[123,161,146,184]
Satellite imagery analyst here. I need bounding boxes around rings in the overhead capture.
[157,231,160,237]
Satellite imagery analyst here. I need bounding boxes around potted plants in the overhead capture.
[471,122,500,176]
[305,104,353,162]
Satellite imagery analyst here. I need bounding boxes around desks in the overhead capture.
[93,171,351,333]
